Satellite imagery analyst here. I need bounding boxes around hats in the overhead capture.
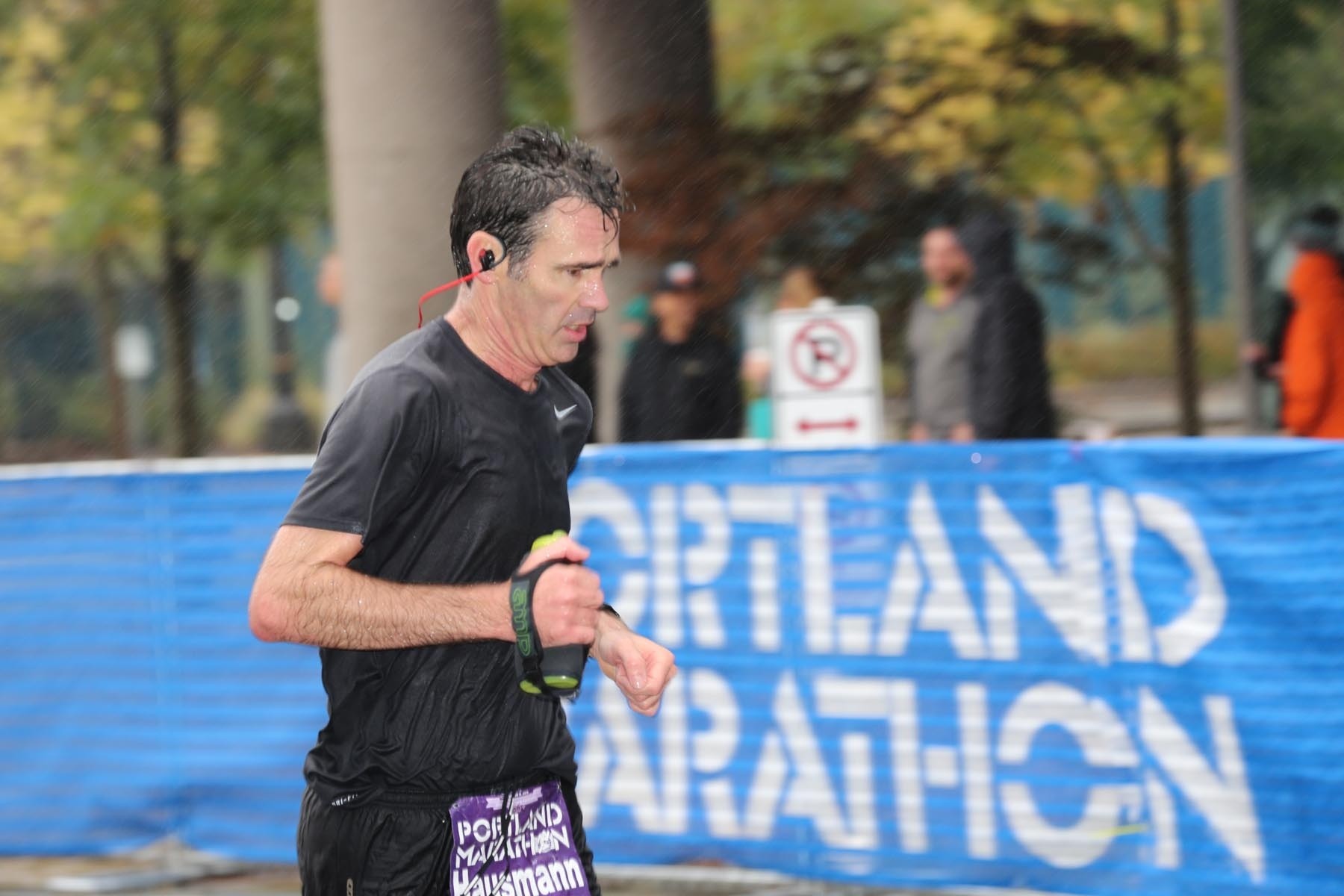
[649,260,704,294]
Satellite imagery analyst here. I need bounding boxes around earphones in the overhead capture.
[478,249,494,271]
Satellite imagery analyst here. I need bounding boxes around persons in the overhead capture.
[617,260,741,443]
[1243,204,1344,439]
[248,124,676,896]
[744,190,1055,441]
